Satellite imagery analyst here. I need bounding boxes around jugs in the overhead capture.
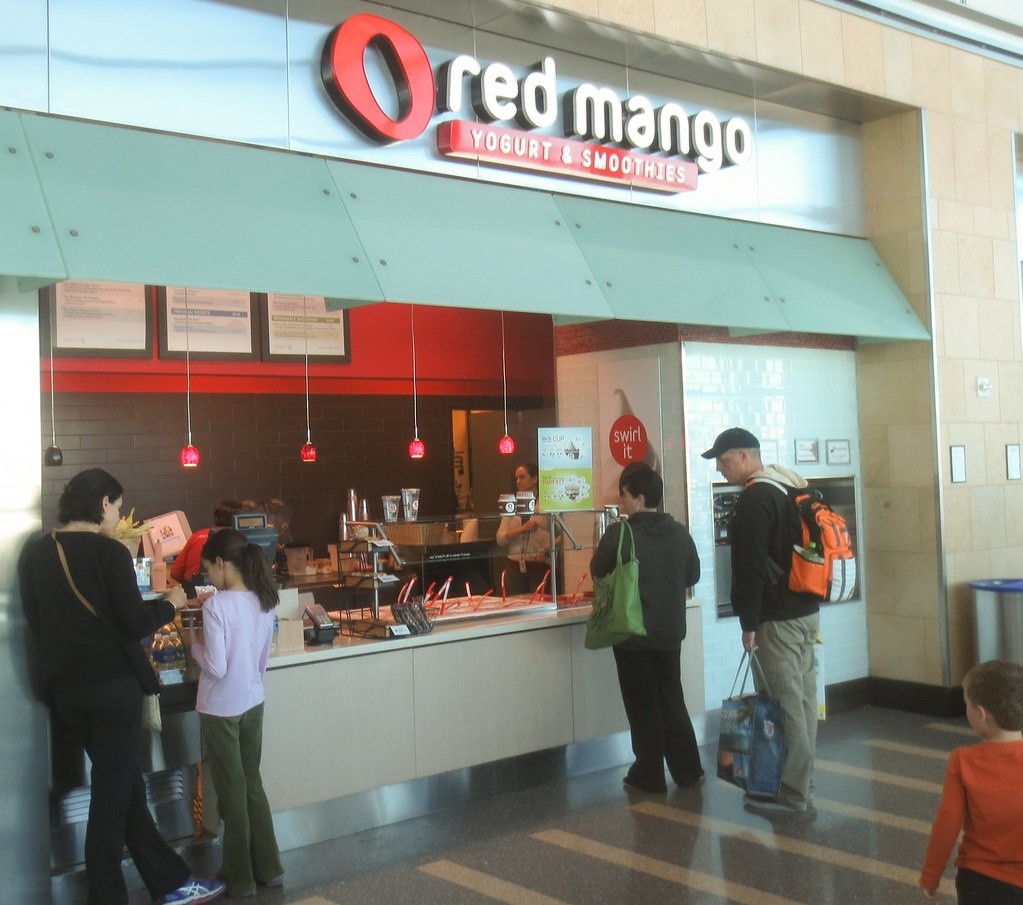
[284,542,313,576]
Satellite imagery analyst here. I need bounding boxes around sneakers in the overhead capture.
[160,875,226,905]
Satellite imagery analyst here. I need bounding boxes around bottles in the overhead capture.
[802,541,819,562]
[150,632,186,679]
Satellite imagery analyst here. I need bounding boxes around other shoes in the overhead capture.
[742,793,807,812]
[623,776,667,797]
[697,768,705,779]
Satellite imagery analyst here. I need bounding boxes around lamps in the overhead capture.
[299,296,317,461]
[180,287,200,466]
[409,304,425,459]
[44,284,63,467]
[498,310,514,454]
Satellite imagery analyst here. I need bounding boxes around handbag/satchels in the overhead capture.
[585,521,648,649]
[716,650,785,793]
[141,695,161,731]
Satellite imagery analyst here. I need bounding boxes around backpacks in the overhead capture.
[743,478,856,603]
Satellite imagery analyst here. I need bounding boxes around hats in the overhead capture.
[700,428,760,459]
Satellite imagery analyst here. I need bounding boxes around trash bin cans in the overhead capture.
[970,579,1023,666]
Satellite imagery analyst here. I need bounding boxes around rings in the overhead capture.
[746,644,748,646]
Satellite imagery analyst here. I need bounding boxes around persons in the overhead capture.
[700,428,819,813]
[588,461,705,792]
[17,467,226,905]
[496,462,562,596]
[919,660,1023,905]
[170,499,243,599]
[189,528,286,898]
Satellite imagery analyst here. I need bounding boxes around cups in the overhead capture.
[347,488,358,522]
[359,499,370,521]
[497,494,518,515]
[382,495,401,523]
[339,513,349,542]
[401,488,420,521]
[516,492,536,514]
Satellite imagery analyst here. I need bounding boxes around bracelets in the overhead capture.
[163,599,177,609]
